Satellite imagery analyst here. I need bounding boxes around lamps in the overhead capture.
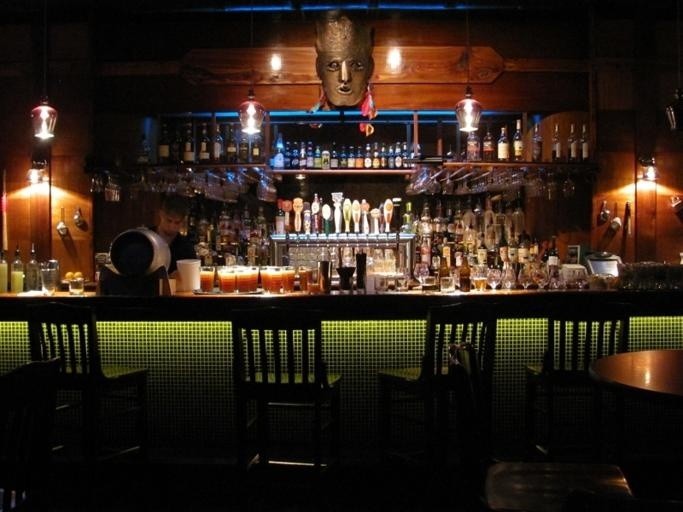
[237,5,264,137]
[23,92,60,142]
[450,11,485,135]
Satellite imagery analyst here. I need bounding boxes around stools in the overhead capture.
[26,302,149,509]
[232,306,343,510]
[521,299,630,464]
[378,301,499,511]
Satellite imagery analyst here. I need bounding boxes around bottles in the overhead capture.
[10,251,23,292]
[24,250,39,293]
[0,253,8,293]
[136,116,592,294]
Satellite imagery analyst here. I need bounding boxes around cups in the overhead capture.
[68,278,84,295]
[38,269,55,296]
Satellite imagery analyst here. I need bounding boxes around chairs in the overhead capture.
[481,461,641,511]
[0,356,62,510]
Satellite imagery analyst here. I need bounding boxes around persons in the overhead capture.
[142,193,197,281]
[306,10,375,138]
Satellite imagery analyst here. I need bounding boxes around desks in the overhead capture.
[584,343,681,508]
